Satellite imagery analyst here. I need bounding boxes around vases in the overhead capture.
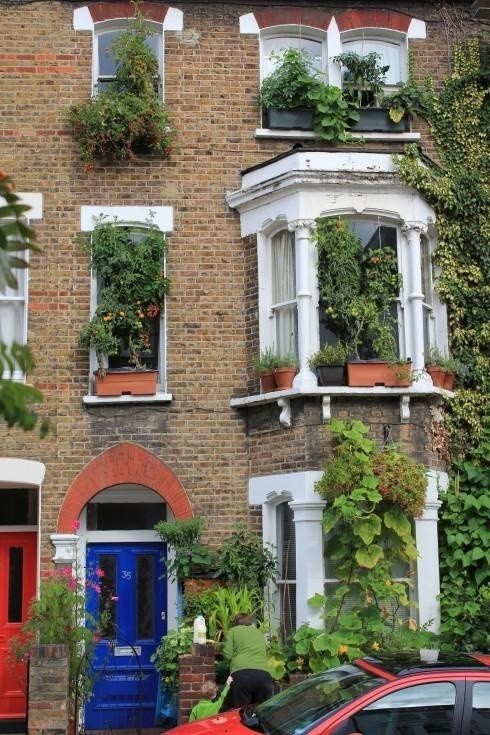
[92,370,161,397]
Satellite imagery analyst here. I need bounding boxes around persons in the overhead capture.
[221,612,275,709]
[187,676,234,721]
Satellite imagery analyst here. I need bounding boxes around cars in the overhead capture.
[163,654,490,735]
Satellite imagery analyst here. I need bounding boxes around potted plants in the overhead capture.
[252,45,390,146]
[253,338,302,395]
[153,518,284,603]
[305,216,413,387]
[423,342,457,391]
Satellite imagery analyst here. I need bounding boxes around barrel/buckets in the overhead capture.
[193,615,207,644]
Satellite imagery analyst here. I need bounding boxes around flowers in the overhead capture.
[4,520,120,706]
[56,1,185,173]
[76,208,172,379]
